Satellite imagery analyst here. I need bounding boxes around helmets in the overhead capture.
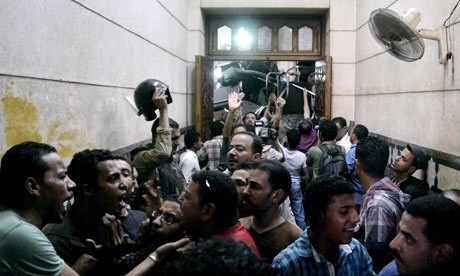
[133,79,174,122]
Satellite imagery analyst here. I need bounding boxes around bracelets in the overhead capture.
[148,253,157,262]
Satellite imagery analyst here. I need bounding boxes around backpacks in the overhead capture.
[317,143,350,178]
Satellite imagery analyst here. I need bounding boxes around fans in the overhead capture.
[367,6,452,69]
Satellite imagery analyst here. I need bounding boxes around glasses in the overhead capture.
[152,209,174,226]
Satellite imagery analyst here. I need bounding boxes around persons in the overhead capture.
[0,88,460,276]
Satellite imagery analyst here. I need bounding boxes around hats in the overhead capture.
[408,142,429,168]
